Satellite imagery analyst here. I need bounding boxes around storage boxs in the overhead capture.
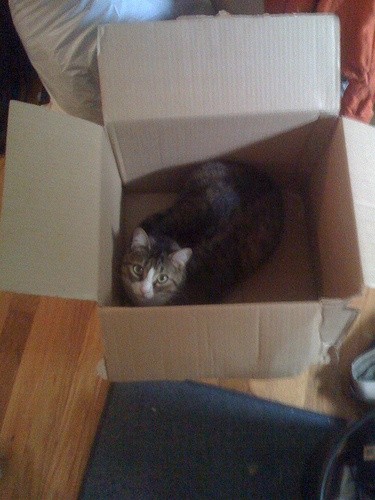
[1,13,375,384]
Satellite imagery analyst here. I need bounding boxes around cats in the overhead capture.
[122,160,283,307]
